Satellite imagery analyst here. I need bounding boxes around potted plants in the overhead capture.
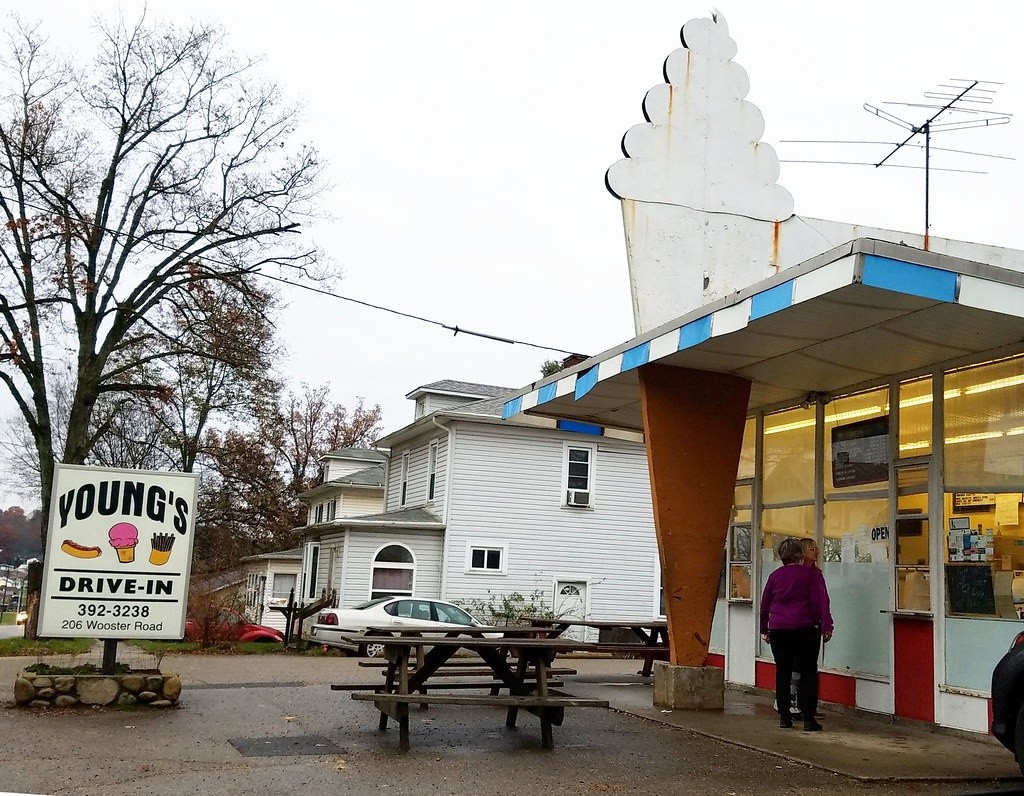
[449,569,607,658]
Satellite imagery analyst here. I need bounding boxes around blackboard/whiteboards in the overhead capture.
[944,562,1001,619]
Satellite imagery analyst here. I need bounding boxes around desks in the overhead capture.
[342,617,669,752]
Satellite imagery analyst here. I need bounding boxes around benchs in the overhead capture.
[596,645,669,653]
[584,642,665,646]
[331,678,565,691]
[358,662,535,667]
[382,667,577,676]
[350,693,610,708]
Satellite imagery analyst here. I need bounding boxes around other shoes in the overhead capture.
[774,699,803,721]
[780,716,793,728]
[804,717,822,731]
[814,712,825,718]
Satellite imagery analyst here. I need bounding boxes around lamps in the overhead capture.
[567,490,590,507]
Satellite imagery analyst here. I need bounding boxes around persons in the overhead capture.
[760,537,834,731]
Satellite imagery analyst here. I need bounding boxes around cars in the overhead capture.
[0,564,29,625]
[300,595,511,658]
[179,606,285,645]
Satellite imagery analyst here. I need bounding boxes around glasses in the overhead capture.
[809,547,820,551]
